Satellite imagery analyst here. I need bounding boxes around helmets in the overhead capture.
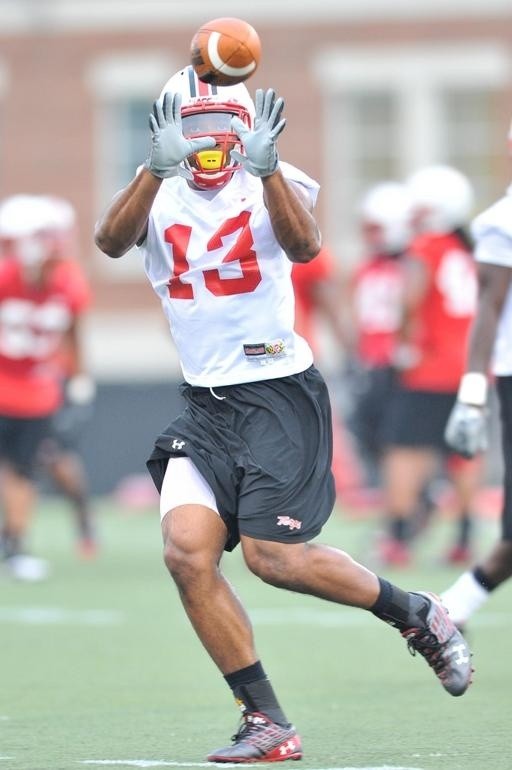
[362,165,473,253]
[1,194,75,241]
[159,66,256,190]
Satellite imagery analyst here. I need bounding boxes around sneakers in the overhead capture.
[207,712,303,762]
[400,591,474,696]
[381,498,472,563]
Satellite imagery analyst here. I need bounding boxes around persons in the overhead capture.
[292,166,512,626]
[1,194,101,579]
[90,64,476,763]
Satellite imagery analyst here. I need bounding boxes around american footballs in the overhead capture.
[191,17,261,86]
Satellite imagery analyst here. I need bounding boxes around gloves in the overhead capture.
[443,401,487,457]
[229,89,286,178]
[148,92,216,180]
